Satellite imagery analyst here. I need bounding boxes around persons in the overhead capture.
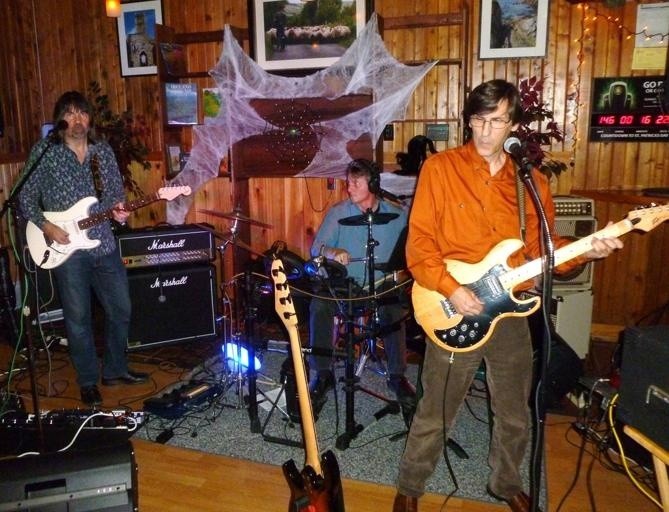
[302,160,416,404]
[18,92,151,408]
[273,6,287,51]
[394,81,624,512]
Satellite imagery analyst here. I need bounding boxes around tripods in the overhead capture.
[389,334,471,459]
[353,229,395,391]
[191,255,295,440]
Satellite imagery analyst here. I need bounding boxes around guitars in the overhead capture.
[266,257,346,511]
[25,182,192,271]
[408,200,669,356]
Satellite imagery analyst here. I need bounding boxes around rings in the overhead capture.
[599,254,604,260]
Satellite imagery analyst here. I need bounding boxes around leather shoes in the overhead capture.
[388,375,416,399]
[308,368,334,395]
[102,369,149,386]
[394,491,418,511]
[80,384,103,405]
[487,484,530,512]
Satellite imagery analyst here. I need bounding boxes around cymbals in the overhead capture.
[198,206,272,230]
[336,212,400,227]
[194,223,265,258]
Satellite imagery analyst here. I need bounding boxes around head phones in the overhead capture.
[346,158,380,192]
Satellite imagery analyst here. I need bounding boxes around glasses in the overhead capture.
[469,117,512,128]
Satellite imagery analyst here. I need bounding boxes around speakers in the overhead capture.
[126,260,216,354]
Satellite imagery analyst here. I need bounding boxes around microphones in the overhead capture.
[45,119,69,139]
[368,181,400,206]
[503,137,533,170]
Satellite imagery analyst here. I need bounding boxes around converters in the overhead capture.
[156,430,174,443]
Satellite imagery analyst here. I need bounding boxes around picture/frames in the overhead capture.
[116,0,165,78]
[247,0,375,77]
[166,143,183,176]
[477,0,550,60]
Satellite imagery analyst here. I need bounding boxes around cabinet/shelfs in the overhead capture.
[153,23,244,180]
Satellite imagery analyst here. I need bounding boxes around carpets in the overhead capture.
[131,333,548,512]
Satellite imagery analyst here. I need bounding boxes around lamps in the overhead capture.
[105,0,121,17]
